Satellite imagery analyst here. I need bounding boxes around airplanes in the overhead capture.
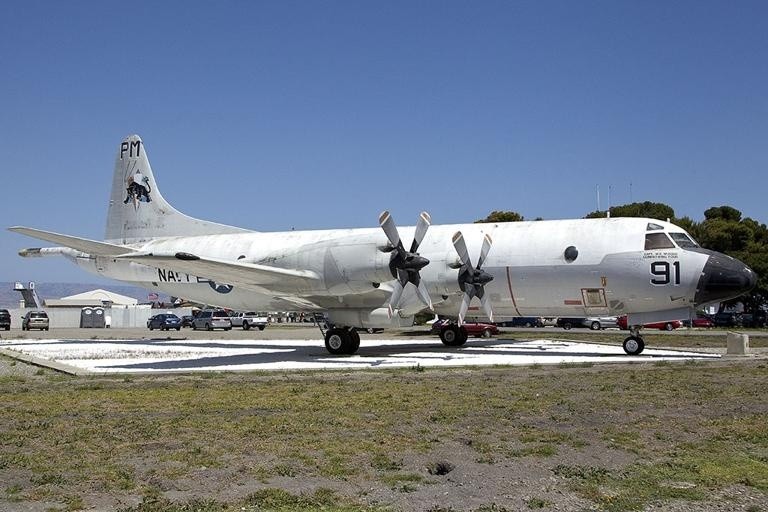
[6,134,759,355]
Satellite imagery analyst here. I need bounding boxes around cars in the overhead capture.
[715,312,737,328]
[431,318,499,337]
[514,314,717,332]
[147,313,195,331]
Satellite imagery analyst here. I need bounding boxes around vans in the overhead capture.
[191,308,232,331]
[21,311,49,331]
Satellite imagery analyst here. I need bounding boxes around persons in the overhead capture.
[277,312,305,323]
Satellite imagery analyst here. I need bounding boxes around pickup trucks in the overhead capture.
[230,312,269,330]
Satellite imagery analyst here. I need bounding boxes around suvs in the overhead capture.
[0,309,10,330]
